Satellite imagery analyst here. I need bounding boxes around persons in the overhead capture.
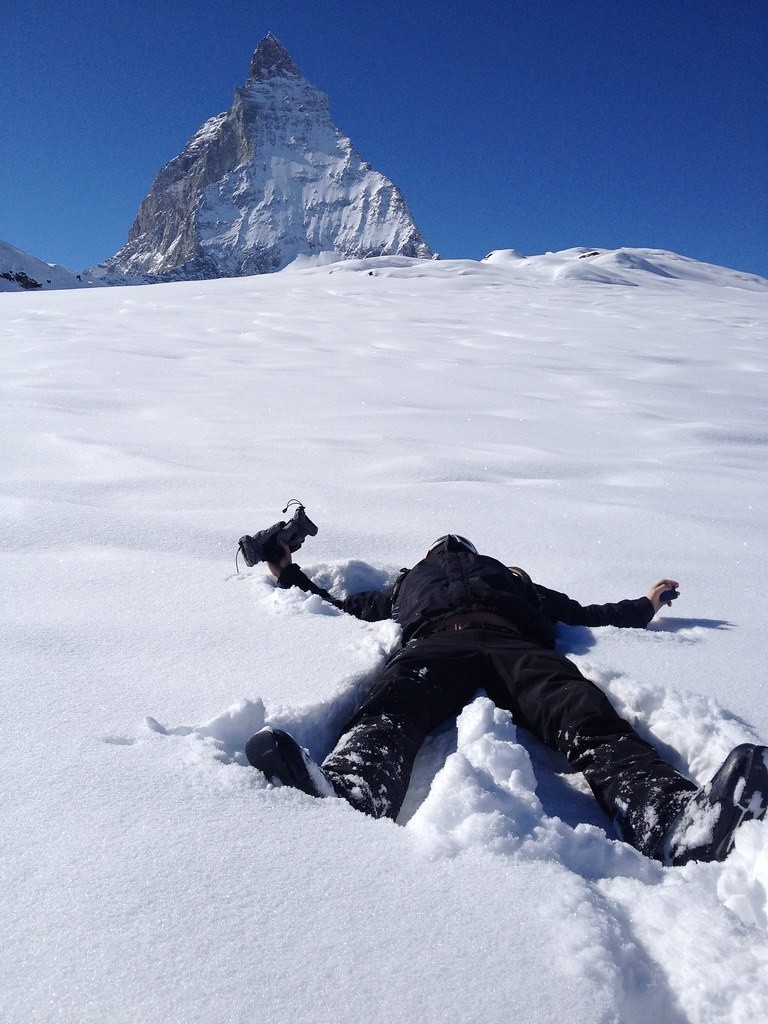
[245,533,768,868]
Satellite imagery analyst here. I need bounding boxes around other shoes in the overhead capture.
[244,730,359,811]
[663,743,768,869]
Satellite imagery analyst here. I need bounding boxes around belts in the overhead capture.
[443,621,514,633]
